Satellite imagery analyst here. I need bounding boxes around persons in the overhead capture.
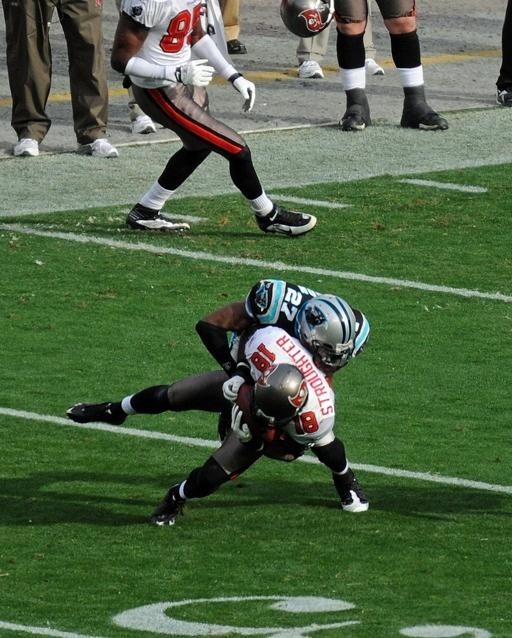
[333,0,448,131]
[495,0,512,107]
[219,0,248,54]
[110,0,317,236]
[115,0,210,135]
[296,0,385,78]
[195,278,371,514]
[2,0,119,158]
[66,324,336,527]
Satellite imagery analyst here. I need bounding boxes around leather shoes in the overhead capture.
[228,39,247,54]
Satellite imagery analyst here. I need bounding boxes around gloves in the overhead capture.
[230,403,253,443]
[222,375,245,402]
[223,66,256,112]
[166,58,215,86]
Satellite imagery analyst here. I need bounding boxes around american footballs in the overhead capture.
[238,382,255,416]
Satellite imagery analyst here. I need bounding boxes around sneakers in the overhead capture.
[14,138,39,157]
[341,103,371,130]
[127,202,191,230]
[132,115,156,134]
[366,58,386,76]
[332,466,369,513]
[493,87,511,106]
[66,402,125,425]
[151,484,183,525]
[79,138,119,158]
[298,60,324,78]
[255,202,317,236]
[400,103,449,130]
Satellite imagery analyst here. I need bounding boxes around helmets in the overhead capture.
[294,294,357,372]
[280,0,336,38]
[254,363,308,430]
